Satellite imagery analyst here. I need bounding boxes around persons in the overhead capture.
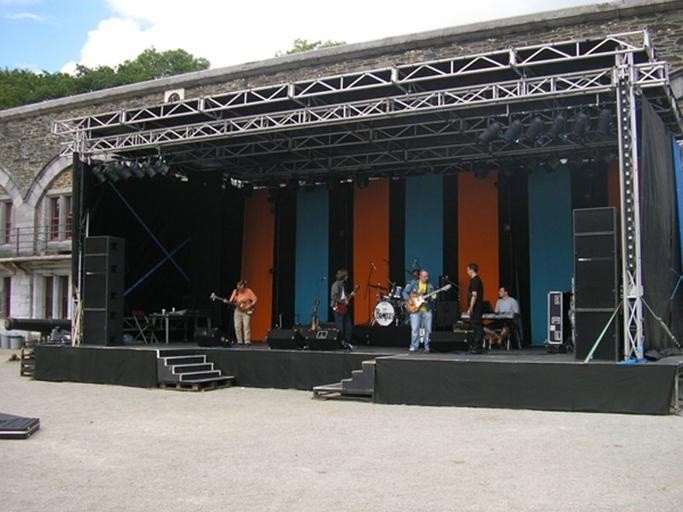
[402,270,439,353]
[405,269,419,288]
[483,286,521,345]
[223,280,258,346]
[330,269,356,348]
[466,263,484,354]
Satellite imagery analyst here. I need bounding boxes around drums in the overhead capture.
[373,301,404,326]
[394,286,403,298]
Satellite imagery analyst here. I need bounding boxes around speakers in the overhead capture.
[194,327,230,346]
[308,330,338,351]
[268,329,308,349]
[83,236,126,346]
[572,206,623,363]
[431,330,465,352]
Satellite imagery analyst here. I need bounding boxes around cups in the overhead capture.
[161,309,165,315]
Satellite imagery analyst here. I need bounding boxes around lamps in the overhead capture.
[85,151,171,182]
[476,103,612,144]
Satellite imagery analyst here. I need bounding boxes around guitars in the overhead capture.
[210,293,256,315]
[404,284,451,313]
[336,284,360,315]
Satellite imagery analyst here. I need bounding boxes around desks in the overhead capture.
[149,314,212,345]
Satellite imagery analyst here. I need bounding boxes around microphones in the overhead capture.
[370,262,376,270]
[320,277,327,280]
[412,259,417,267]
[445,273,448,282]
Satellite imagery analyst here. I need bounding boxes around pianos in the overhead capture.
[460,312,519,328]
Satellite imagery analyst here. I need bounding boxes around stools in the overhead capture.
[487,326,521,353]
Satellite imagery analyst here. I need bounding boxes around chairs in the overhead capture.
[132,310,158,345]
